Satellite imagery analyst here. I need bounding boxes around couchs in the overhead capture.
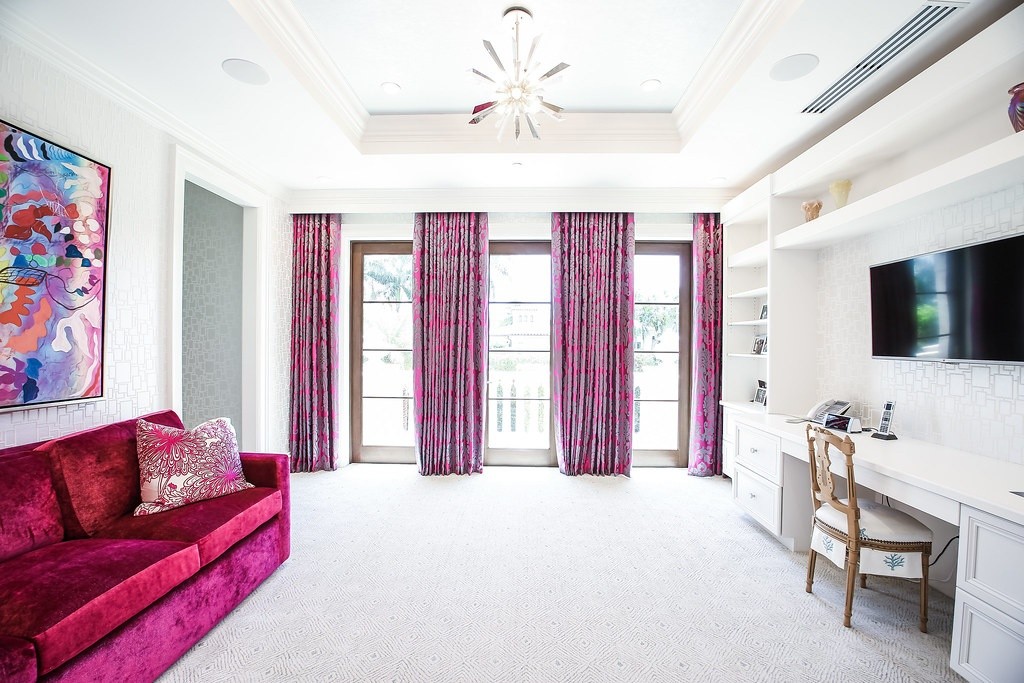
[0,408,289,683]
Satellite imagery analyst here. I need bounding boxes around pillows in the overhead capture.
[134,418,255,516]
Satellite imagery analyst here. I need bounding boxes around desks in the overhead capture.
[736,413,1024,682]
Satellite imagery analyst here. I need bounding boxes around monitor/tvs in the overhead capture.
[870,232,1024,366]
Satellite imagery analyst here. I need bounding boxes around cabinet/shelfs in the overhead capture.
[720,7,1024,482]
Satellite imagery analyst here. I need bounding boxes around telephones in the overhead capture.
[805,398,852,424]
[870,399,898,440]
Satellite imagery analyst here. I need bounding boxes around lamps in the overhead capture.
[465,8,573,144]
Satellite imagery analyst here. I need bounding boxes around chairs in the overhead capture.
[803,425,932,635]
